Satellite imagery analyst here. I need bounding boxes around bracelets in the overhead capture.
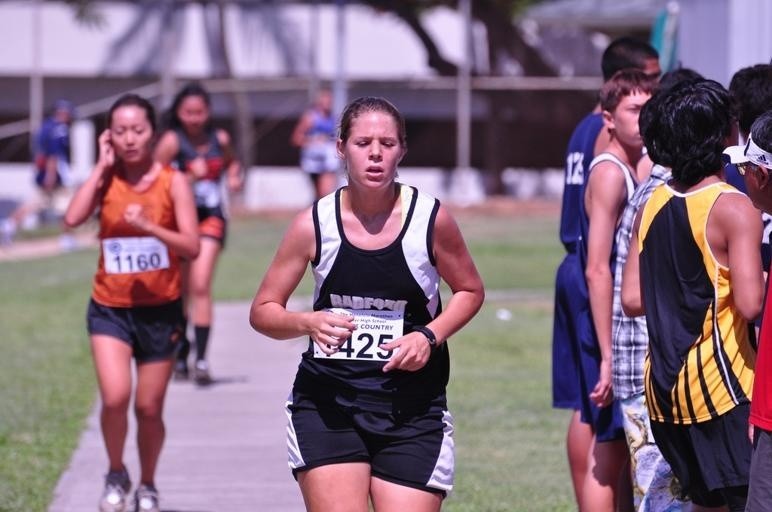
[411,324,436,353]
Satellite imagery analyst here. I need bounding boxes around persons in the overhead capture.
[64,94,200,512]
[620,77,766,512]
[249,96,484,512]
[9,100,93,237]
[722,110,772,512]
[582,67,660,512]
[152,81,244,385]
[611,89,693,512]
[723,64,772,146]
[551,35,663,512]
[291,81,344,196]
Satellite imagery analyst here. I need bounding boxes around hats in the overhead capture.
[723,131,771,169]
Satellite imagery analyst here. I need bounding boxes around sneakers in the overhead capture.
[174,361,187,379]
[136,484,159,512]
[195,360,211,384]
[100,464,131,512]
